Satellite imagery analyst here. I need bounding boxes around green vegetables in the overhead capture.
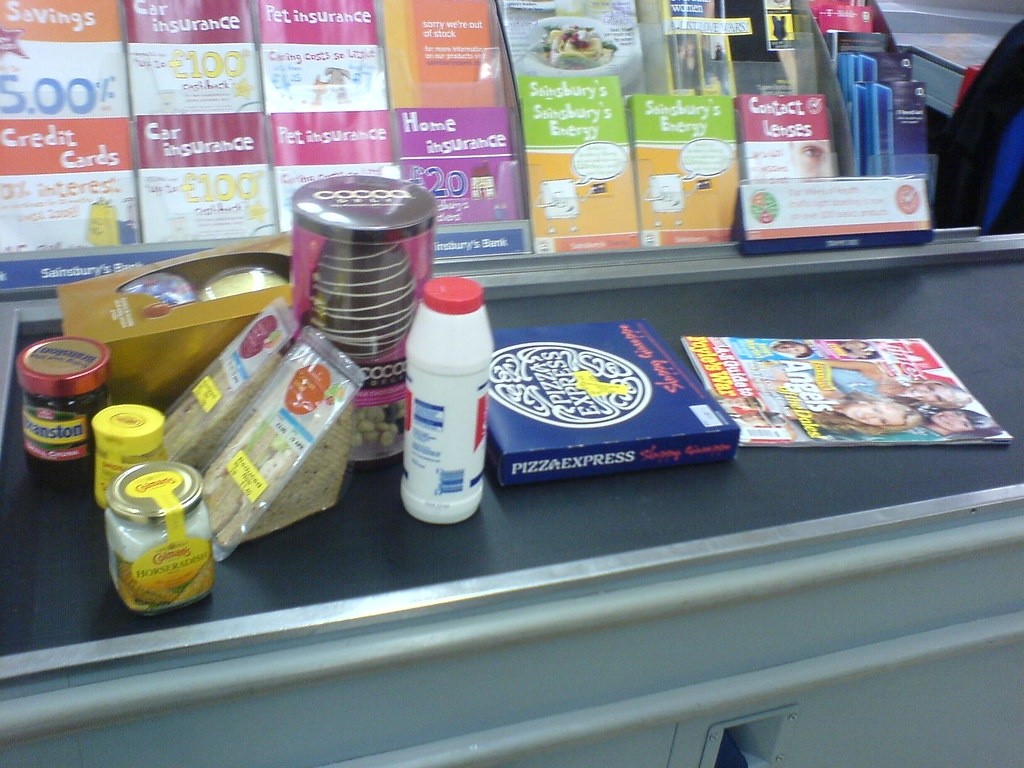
[540,23,618,52]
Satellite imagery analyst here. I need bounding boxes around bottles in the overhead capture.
[400,276,494,524]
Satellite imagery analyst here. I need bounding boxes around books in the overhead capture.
[681,334,1014,451]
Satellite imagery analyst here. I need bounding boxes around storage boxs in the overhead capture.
[55,230,294,415]
[486,318,742,489]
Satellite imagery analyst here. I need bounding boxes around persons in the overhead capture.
[774,339,1004,444]
[928,17,1024,236]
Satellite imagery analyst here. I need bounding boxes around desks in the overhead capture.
[0,233,1024,768]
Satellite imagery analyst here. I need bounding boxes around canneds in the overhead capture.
[16,335,110,490]
[101,461,214,614]
[91,403,170,506]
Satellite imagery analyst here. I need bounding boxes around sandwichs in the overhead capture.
[154,338,353,551]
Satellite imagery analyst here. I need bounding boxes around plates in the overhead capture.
[527,16,629,77]
